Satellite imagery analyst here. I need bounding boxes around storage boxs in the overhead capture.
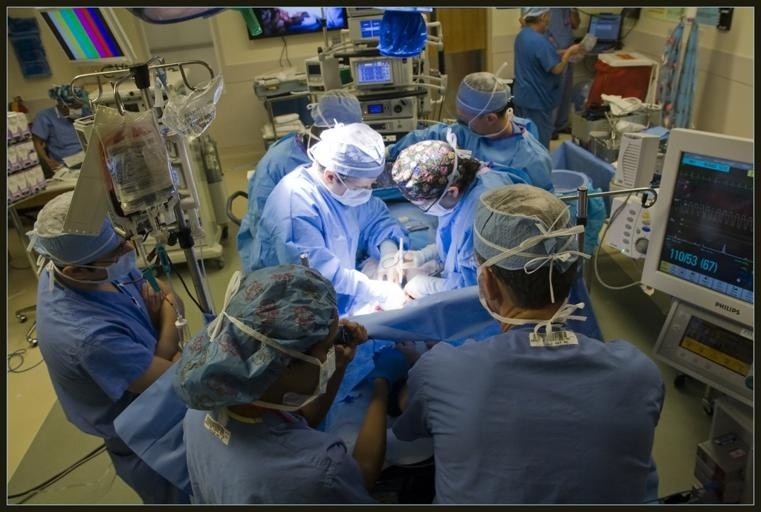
[585,51,656,109]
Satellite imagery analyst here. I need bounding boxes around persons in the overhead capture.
[179,259,391,503]
[514,5,588,148]
[396,182,667,504]
[26,189,185,504]
[236,72,600,341]
[545,8,583,142]
[32,85,88,181]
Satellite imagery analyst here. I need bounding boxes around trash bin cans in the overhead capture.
[551,169,592,206]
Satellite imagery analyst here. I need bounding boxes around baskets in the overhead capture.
[572,102,663,143]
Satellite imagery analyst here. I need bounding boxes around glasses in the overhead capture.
[413,194,442,215]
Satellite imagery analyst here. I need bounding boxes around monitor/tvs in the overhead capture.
[640,126,754,329]
[245,7,349,39]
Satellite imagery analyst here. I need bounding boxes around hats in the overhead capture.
[474,183,580,273]
[312,123,387,177]
[391,140,465,202]
[25,192,120,266]
[174,263,338,410]
[455,71,512,114]
[48,83,89,104]
[310,90,363,127]
[520,7,549,18]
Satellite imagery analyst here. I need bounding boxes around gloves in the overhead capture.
[395,243,439,271]
[338,270,407,313]
[404,275,453,299]
[369,343,410,385]
[376,241,406,286]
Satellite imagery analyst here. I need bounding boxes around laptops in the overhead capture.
[569,12,624,53]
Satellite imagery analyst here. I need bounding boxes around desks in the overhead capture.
[8,175,79,283]
[262,88,310,142]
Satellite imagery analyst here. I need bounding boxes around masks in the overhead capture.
[317,349,337,398]
[68,108,82,120]
[106,250,137,282]
[333,190,373,207]
[468,125,484,141]
[423,205,452,217]
